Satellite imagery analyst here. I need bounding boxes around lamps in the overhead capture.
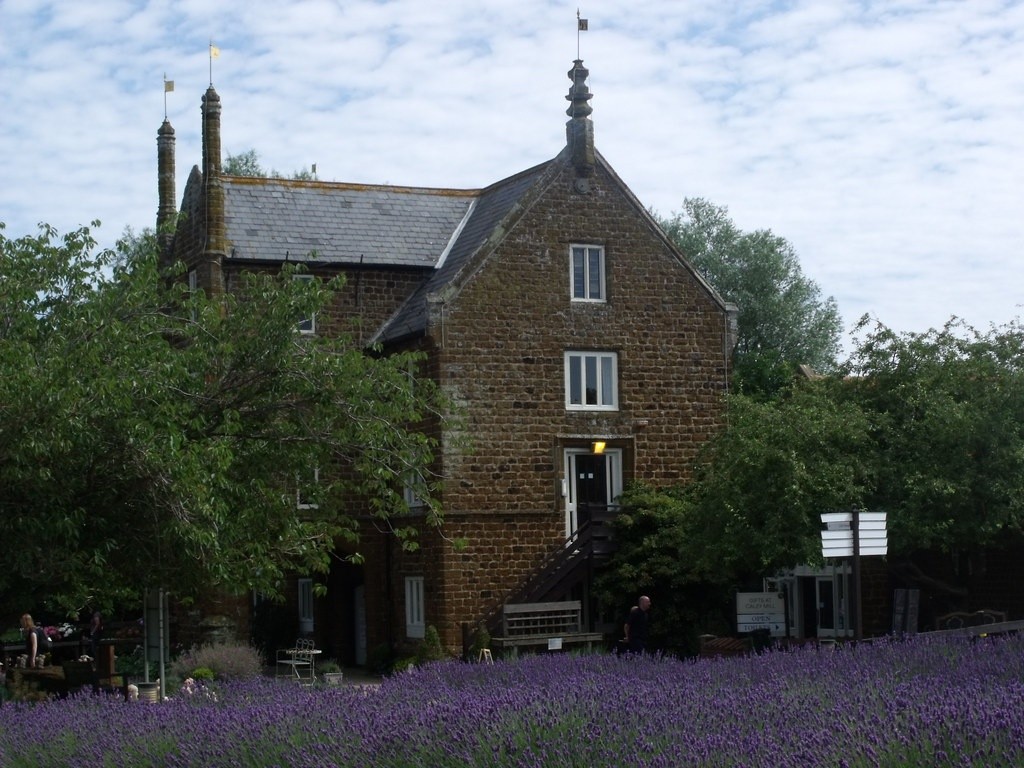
[590,441,607,454]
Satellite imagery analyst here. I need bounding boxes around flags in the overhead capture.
[211,44,220,58]
[166,81,174,92]
[580,19,588,30]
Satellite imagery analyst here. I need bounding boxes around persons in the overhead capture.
[89,604,103,660]
[625,596,651,652]
[21,613,50,669]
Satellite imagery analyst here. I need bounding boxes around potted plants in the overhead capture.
[321,662,343,686]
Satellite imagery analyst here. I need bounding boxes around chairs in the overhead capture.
[56,651,129,701]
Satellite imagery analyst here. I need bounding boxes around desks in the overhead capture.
[286,649,322,686]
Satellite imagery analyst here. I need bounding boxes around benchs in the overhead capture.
[935,610,1007,637]
[490,600,604,658]
[698,632,836,657]
[275,638,317,689]
